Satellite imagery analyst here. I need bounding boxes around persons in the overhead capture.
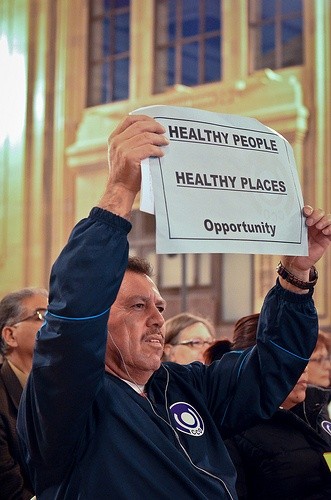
[0,289,49,499]
[161,313,215,365]
[203,313,331,499]
[16,114,331,500]
[306,332,331,389]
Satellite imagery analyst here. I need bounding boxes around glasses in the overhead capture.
[10,308,46,327]
[171,340,213,350]
[310,355,331,365]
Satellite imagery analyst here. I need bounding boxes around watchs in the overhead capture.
[276,261,318,289]
